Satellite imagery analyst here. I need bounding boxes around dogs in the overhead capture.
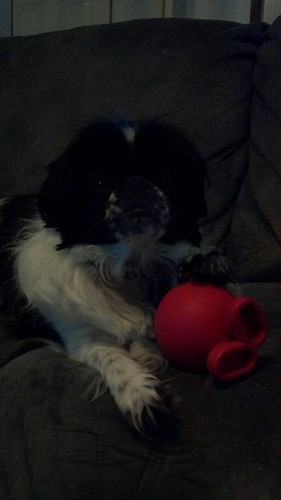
[0,116,241,432]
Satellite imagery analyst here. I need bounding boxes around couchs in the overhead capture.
[1,11,281,498]
